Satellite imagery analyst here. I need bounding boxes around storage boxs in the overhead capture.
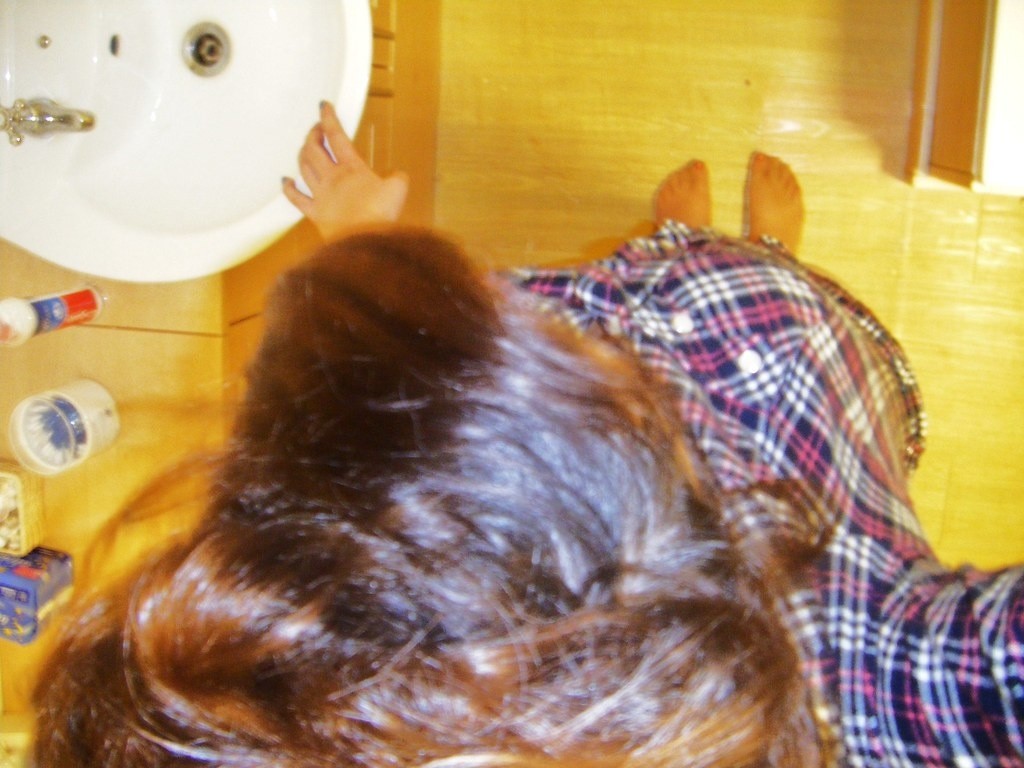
[0,547,74,643]
[0,461,46,556]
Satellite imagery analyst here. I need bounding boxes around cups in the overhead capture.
[7,381,119,476]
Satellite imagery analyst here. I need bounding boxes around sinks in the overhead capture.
[1,1,375,284]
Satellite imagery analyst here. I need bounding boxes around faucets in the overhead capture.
[0,97,95,146]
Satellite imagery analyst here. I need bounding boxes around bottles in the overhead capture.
[1,284,102,344]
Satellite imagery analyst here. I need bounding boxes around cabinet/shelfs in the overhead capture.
[226,0,440,429]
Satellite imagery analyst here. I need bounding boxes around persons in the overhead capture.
[33,102,1024,768]
